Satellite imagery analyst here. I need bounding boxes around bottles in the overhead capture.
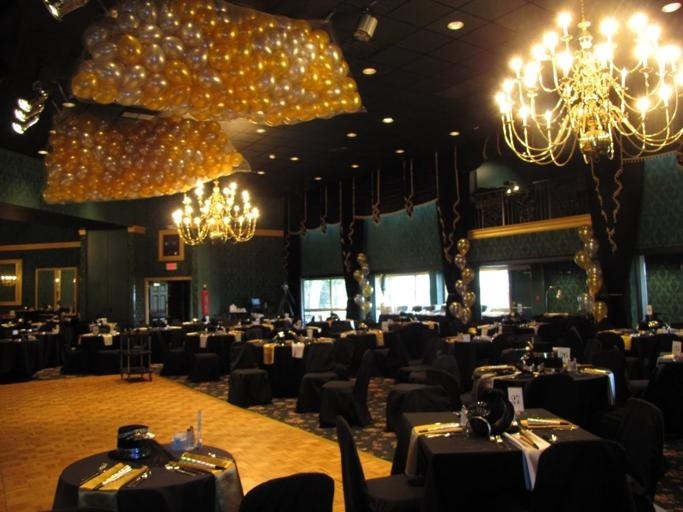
[460,405,467,427]
[187,424,194,448]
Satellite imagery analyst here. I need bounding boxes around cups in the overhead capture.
[306,330,313,339]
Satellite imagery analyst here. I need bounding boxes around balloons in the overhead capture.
[353,253,373,319]
[448,238,477,326]
[573,222,609,323]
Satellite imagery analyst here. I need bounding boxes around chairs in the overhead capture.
[616,399,663,512]
[530,439,634,511]
[236,472,333,512]
[334,412,426,511]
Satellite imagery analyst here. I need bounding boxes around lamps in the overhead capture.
[172,180,262,246]
[353,14,379,42]
[466,193,594,241]
[491,2,683,168]
[11,82,48,135]
